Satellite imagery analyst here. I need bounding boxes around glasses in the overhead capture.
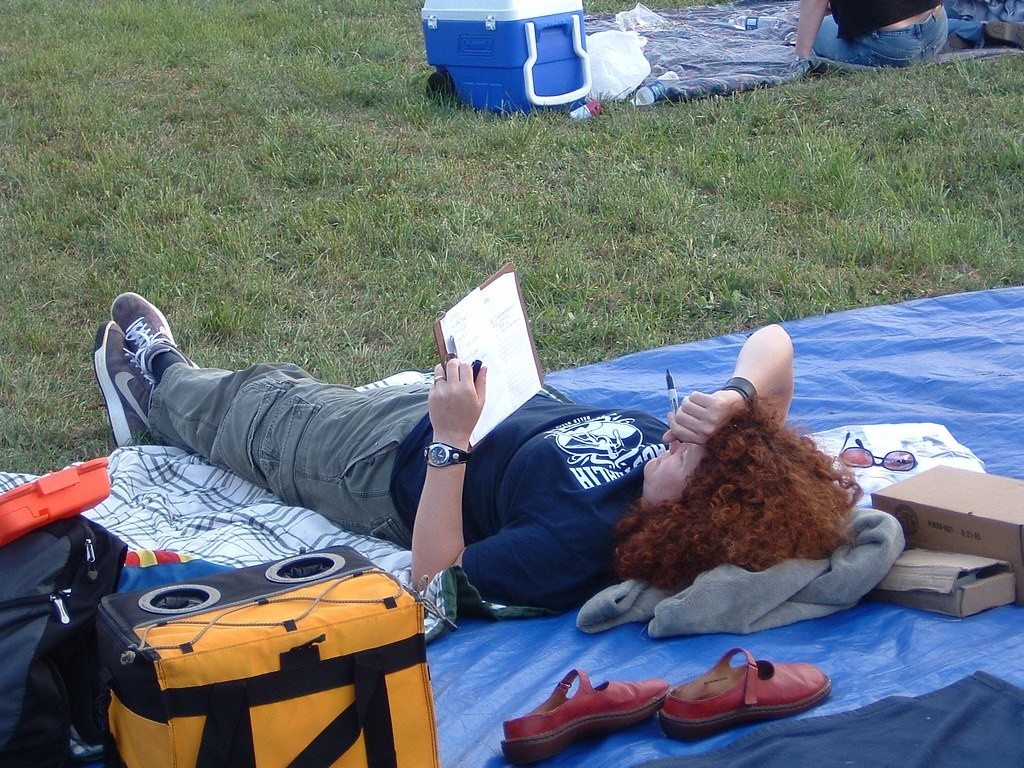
[838,432,918,472]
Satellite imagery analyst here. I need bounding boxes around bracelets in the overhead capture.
[722,377,758,407]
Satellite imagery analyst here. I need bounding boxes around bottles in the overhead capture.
[630,71,679,106]
[729,16,780,29]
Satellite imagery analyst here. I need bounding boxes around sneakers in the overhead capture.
[110,292,201,374]
[93,320,157,449]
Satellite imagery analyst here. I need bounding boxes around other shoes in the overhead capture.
[659,646,832,742]
[500,668,671,765]
[938,32,975,54]
[983,21,1024,48]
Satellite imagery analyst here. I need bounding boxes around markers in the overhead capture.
[666,369,683,443]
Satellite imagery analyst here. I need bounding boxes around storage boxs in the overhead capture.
[871,466,1024,608]
[861,548,1016,618]
[421,0,592,116]
[0,457,111,548]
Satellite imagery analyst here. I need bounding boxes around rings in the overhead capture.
[434,375,446,384]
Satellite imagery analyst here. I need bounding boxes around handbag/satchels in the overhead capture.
[96,544,441,768]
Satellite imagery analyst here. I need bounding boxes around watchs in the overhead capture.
[794,55,807,62]
[423,442,470,467]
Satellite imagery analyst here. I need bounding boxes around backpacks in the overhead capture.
[0,513,128,768]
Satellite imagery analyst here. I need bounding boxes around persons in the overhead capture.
[93,292,863,607]
[792,0,1024,74]
